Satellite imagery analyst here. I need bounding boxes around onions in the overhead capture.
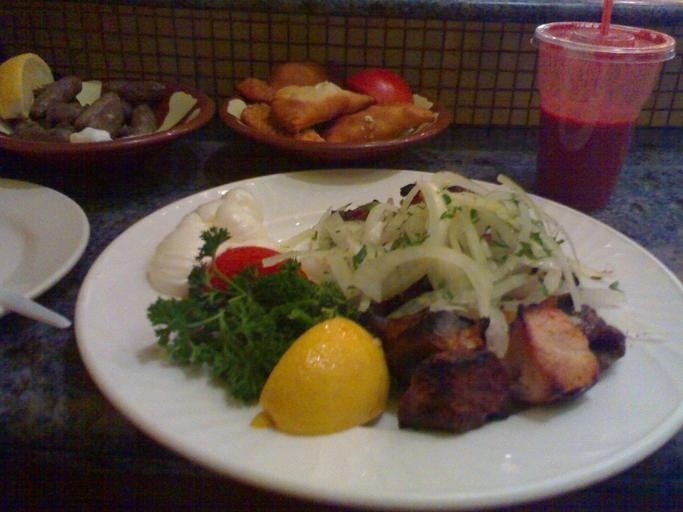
[259,168,628,359]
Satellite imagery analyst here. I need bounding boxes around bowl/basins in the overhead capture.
[0,83,215,187]
[218,90,452,172]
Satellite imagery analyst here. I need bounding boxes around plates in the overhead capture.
[73,169,683,508]
[1,178,90,334]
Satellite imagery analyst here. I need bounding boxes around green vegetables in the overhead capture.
[145,227,353,406]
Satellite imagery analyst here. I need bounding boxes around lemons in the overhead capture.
[250,316,391,437]
[0,52,56,120]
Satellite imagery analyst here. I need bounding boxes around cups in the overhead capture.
[530,24,675,214]
